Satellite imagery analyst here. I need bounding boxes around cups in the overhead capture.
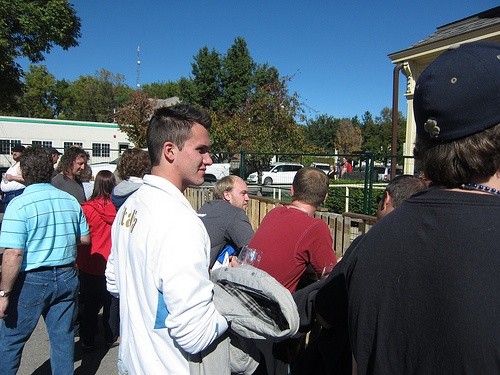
[2,173,7,180]
[235,244,263,270]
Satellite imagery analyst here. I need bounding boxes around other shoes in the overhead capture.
[81,338,94,351]
[105,336,119,346]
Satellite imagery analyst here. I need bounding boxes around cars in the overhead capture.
[205,154,230,181]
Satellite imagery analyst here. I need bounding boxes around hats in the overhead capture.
[412,40,500,147]
[47,147,62,155]
[13,146,27,152]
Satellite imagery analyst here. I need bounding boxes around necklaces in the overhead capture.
[462,182,500,196]
[289,203,308,214]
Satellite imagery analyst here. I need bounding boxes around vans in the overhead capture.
[374,164,404,181]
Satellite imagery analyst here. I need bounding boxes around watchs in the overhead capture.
[0,289,11,299]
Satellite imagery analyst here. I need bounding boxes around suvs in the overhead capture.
[310,162,332,177]
[246,162,306,186]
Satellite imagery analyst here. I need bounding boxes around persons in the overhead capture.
[0,43,500,375]
[302,51,500,375]
[105,103,230,375]
[0,147,91,375]
